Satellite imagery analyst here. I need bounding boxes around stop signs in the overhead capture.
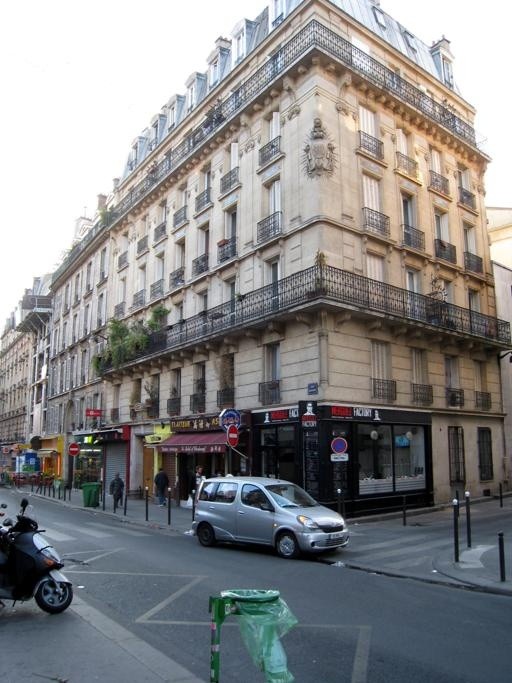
[69,442,80,456]
[227,423,239,447]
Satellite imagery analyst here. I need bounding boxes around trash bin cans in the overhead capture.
[81,482,102,507]
[208,589,298,683]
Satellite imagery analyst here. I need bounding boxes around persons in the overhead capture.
[155,467,169,507]
[302,402,317,426]
[188,464,204,499]
[109,471,124,508]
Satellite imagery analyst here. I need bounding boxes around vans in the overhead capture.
[193,474,350,561]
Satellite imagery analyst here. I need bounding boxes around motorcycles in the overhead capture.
[0,496,74,614]
